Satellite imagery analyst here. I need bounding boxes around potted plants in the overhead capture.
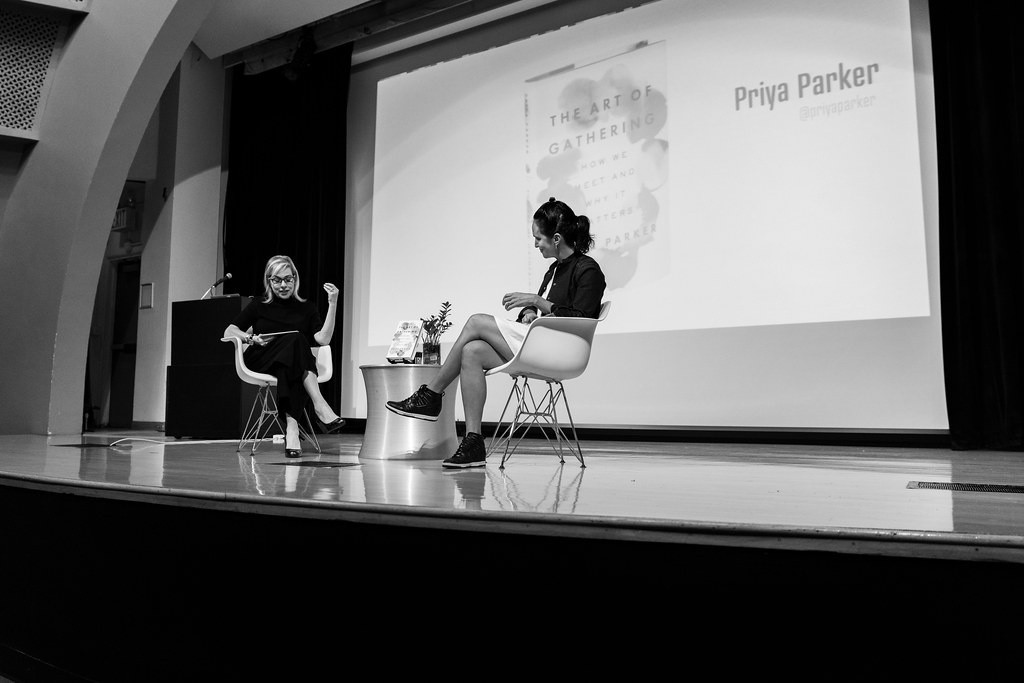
[420,301,452,365]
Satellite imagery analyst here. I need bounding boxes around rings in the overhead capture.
[329,288,334,293]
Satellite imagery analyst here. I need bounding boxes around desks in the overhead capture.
[359,364,460,460]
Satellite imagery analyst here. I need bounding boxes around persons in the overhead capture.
[385,197,606,468]
[224,255,346,458]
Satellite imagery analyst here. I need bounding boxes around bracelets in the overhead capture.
[247,334,254,346]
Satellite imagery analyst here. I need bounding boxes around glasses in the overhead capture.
[269,275,296,284]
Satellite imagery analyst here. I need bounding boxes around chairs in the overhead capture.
[221,335,333,456]
[482,301,611,468]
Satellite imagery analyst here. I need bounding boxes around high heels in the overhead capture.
[284,429,302,457]
[315,411,346,433]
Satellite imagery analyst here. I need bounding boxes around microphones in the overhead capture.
[210,273,232,289]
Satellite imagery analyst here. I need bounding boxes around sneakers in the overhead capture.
[442,432,486,467]
[385,384,445,421]
